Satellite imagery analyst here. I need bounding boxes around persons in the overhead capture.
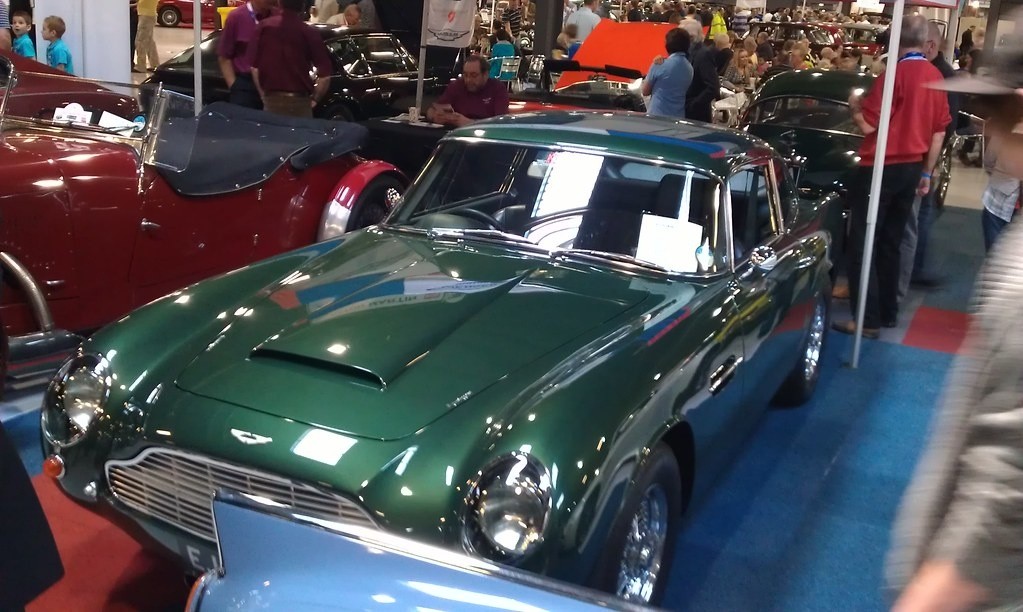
[310,0,339,23]
[768,8,890,71]
[325,4,361,26]
[130,0,159,73]
[641,29,694,118]
[830,13,953,340]
[895,20,959,298]
[620,0,774,93]
[473,0,602,92]
[11,11,36,61]
[41,14,74,76]
[954,48,982,76]
[426,56,510,127]
[214,0,332,119]
[678,18,721,123]
[203,0,228,32]
[960,25,976,51]
[880,101,1023,612]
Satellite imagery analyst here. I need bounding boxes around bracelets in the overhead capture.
[920,172,931,178]
[309,93,321,103]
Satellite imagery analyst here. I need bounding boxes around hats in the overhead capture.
[922,7,1023,94]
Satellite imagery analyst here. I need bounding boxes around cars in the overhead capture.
[36,105,854,610]
[0,1,956,395]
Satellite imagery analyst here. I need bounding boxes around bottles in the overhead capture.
[409,105,418,123]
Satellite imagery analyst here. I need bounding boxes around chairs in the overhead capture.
[651,175,718,239]
[486,56,521,91]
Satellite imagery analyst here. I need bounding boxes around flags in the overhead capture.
[426,0,476,47]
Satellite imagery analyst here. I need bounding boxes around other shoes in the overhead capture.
[880,319,896,328]
[830,283,849,298]
[832,320,879,338]
